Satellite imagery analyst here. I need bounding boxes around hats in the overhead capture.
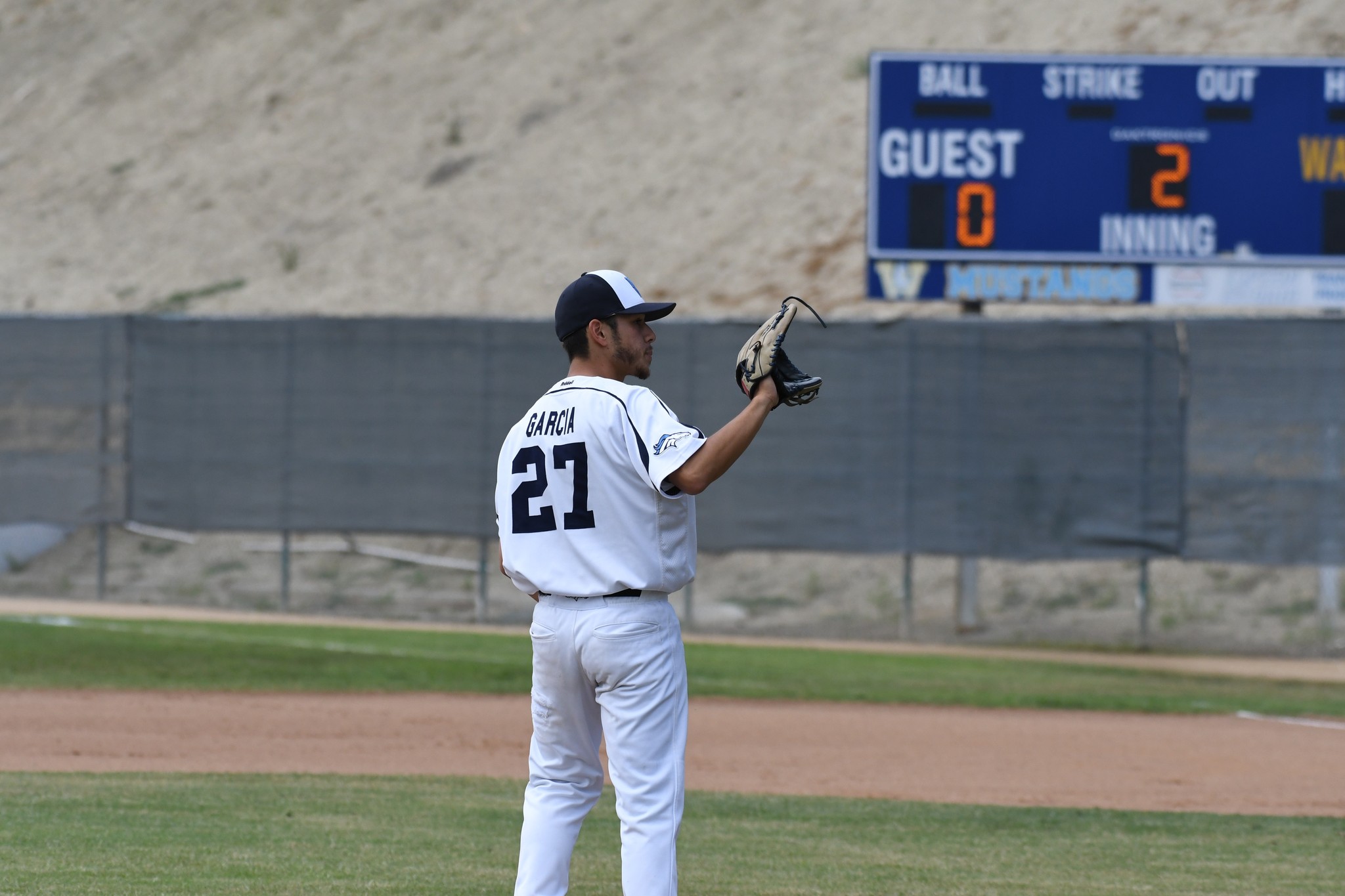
[554,269,676,342]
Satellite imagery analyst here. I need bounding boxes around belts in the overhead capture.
[538,588,642,598]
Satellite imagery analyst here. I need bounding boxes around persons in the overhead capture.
[495,268,824,895]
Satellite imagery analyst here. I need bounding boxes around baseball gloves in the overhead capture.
[735,303,824,412]
